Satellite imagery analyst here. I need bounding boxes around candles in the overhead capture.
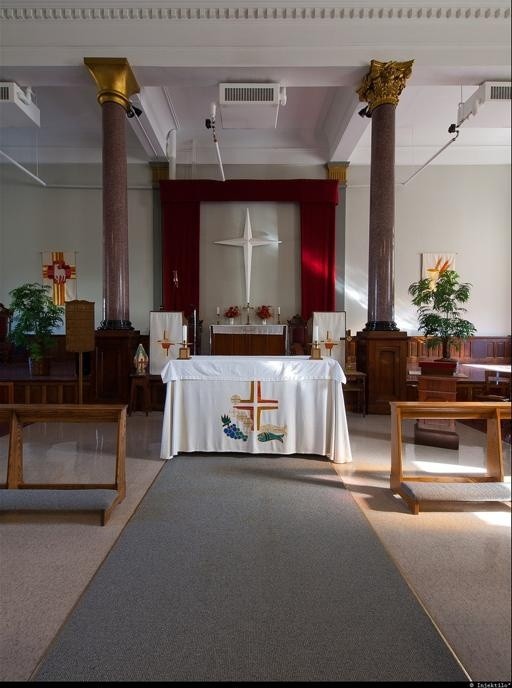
[182,325,187,343]
[313,325,318,344]
[217,307,219,314]
[278,307,280,314]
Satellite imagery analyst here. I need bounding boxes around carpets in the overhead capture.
[29,456,471,680]
[0,404,167,681]
[333,409,512,681]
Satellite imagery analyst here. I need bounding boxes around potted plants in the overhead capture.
[7,282,64,375]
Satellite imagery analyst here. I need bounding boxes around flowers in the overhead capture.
[224,306,240,318]
[256,305,273,320]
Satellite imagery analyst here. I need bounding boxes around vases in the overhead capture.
[229,318,234,324]
[262,319,266,325]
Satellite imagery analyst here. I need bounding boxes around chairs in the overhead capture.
[342,369,367,418]
[477,371,512,402]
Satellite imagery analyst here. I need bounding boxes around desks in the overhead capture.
[160,355,352,464]
[209,325,288,354]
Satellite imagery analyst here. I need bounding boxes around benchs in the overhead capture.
[129,374,152,417]
